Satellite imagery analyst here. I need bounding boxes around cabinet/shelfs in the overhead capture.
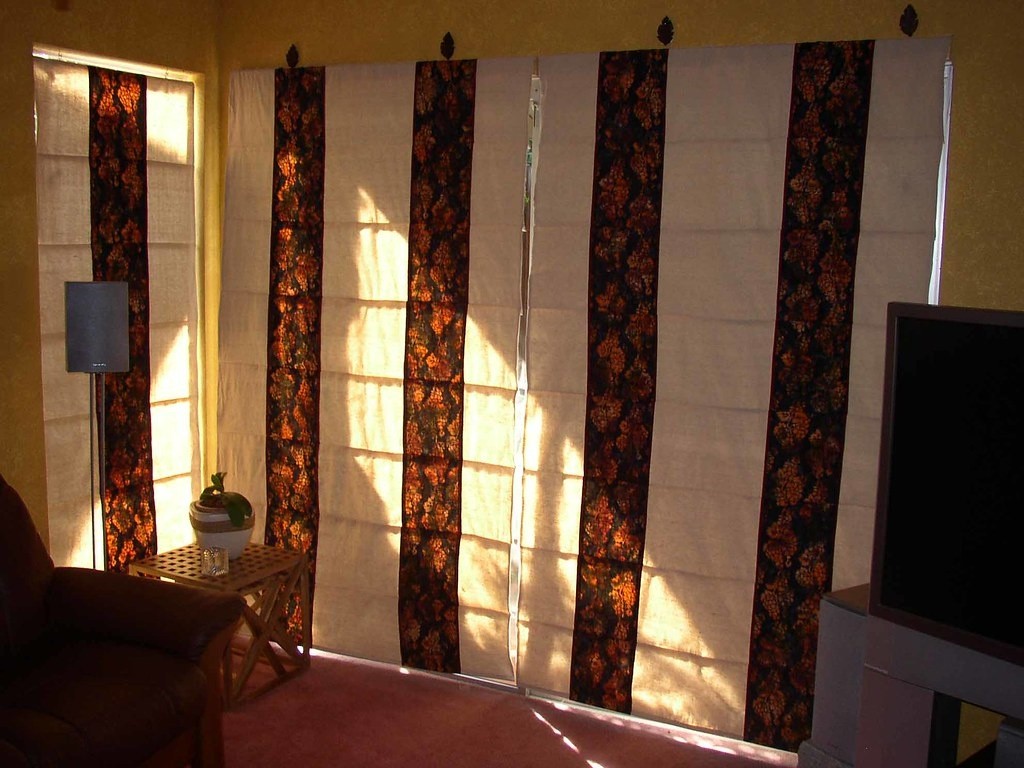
[797,583,1024,768]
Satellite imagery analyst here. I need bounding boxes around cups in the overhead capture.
[201,548,229,576]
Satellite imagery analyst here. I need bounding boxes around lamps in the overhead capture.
[61,280,131,570]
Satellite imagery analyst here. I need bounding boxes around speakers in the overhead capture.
[63,281,129,374]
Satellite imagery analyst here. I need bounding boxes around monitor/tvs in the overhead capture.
[869,301,1024,668]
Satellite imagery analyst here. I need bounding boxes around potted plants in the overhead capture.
[189,473,255,560]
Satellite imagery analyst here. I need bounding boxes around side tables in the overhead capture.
[129,540,312,709]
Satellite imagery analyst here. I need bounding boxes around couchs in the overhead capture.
[0,473,249,768]
